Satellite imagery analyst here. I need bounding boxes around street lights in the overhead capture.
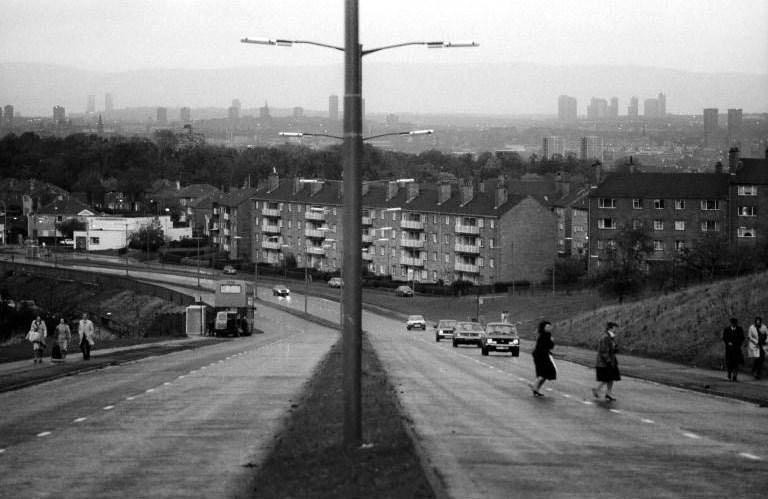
[240,36,479,104]
[543,195,559,296]
[282,244,308,314]
[53,206,59,262]
[114,222,138,276]
[86,227,103,260]
[277,128,436,141]
[188,236,206,288]
[299,177,416,269]
[233,235,278,299]
[92,312,112,341]
[164,208,170,252]
[1,200,8,246]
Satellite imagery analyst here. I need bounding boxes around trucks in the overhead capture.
[205,280,257,337]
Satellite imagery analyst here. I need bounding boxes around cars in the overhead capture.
[328,277,344,289]
[483,322,521,356]
[223,264,237,275]
[395,285,414,297]
[407,313,482,349]
[59,238,74,246]
[272,283,290,297]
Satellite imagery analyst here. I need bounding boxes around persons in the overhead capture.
[528,321,557,399]
[53,317,73,360]
[77,312,96,361]
[591,321,622,403]
[746,316,767,380]
[24,315,48,364]
[722,316,745,381]
[502,310,511,324]
[500,310,505,323]
